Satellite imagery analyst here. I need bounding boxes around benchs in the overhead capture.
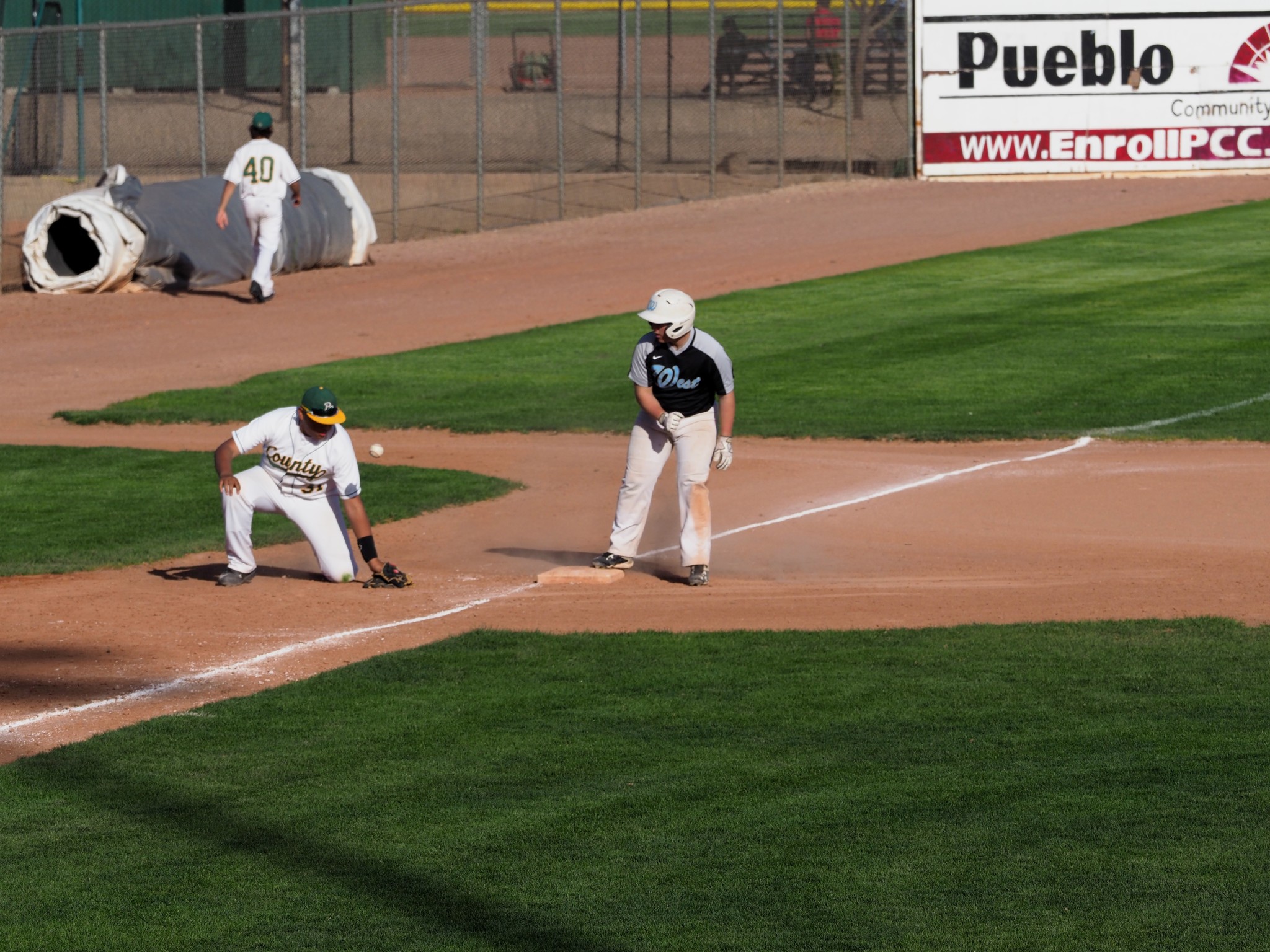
[718,13,908,98]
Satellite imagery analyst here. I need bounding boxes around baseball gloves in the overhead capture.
[362,561,413,590]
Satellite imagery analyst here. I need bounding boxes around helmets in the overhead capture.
[637,288,696,340]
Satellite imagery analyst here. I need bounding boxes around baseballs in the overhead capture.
[368,443,385,458]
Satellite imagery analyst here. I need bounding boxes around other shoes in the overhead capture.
[250,281,275,302]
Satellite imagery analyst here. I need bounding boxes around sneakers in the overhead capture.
[591,551,634,570]
[688,564,709,585]
[219,564,256,586]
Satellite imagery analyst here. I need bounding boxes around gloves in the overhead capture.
[658,411,684,433]
[713,435,734,470]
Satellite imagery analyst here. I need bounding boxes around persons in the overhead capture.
[216,110,303,303]
[802,0,841,103]
[701,17,747,95]
[592,288,734,586]
[214,387,414,588]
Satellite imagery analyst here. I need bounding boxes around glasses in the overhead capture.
[304,407,338,418]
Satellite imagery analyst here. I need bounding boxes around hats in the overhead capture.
[253,112,272,129]
[301,386,346,425]
